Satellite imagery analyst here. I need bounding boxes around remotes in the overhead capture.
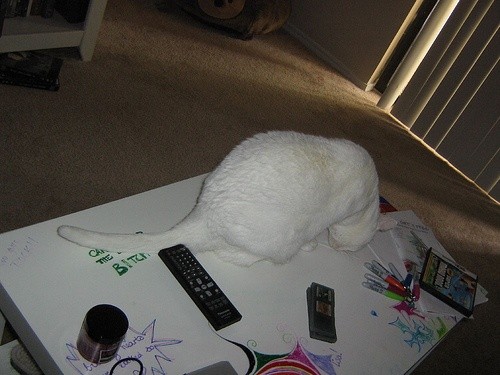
[158,243,243,332]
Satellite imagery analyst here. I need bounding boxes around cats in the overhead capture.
[56,130,398,267]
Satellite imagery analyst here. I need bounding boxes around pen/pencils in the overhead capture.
[362,259,421,306]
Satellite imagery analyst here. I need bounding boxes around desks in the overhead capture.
[1,172,488,375]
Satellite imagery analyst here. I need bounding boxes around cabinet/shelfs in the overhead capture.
[0,0,107,63]
